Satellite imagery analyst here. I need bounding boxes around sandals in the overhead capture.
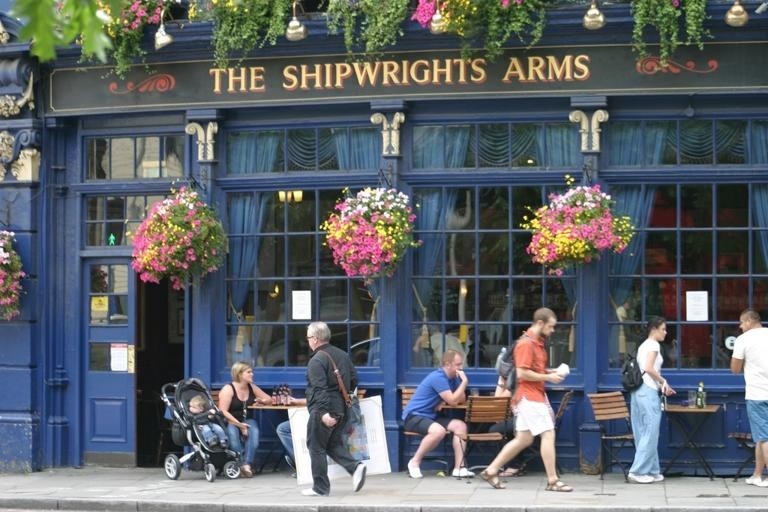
[479,469,506,488]
[545,479,573,492]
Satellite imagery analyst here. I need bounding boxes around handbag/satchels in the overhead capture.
[345,394,362,427]
[496,351,518,392]
[621,353,642,390]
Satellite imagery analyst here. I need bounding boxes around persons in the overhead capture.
[464,375,525,477]
[276,396,307,477]
[218,362,272,478]
[401,349,476,478]
[189,395,228,449]
[301,320,367,497]
[730,308,768,487]
[627,315,677,484]
[413,325,486,369]
[480,307,574,492]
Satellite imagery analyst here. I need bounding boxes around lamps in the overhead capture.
[583,0,607,30]
[725,0,748,27]
[429,0,449,34]
[286,0,308,42]
[154,8,175,51]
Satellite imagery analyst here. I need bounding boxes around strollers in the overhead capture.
[158,375,241,484]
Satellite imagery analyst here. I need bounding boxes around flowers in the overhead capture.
[412,0,561,64]
[57,0,164,78]
[188,0,292,73]
[320,187,425,285]
[520,184,637,277]
[130,181,228,291]
[0,231,28,319]
[629,0,715,66]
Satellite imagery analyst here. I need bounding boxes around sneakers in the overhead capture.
[407,458,423,479]
[210,439,227,448]
[241,463,253,477]
[301,487,330,497]
[627,471,665,483]
[352,463,367,492]
[452,466,475,477]
[745,475,768,487]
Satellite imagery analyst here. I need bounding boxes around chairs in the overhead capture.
[587,391,634,482]
[401,388,575,484]
[210,391,220,409]
[356,390,366,400]
[727,431,755,481]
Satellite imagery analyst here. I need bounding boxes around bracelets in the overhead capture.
[497,383,505,388]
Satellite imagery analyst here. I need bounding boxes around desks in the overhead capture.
[664,405,721,481]
[247,404,306,474]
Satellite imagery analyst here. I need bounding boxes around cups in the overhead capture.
[687,390,697,409]
[470,388,479,396]
[679,400,689,407]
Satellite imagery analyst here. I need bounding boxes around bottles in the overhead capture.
[696,381,705,409]
[489,390,494,396]
[270,383,291,407]
